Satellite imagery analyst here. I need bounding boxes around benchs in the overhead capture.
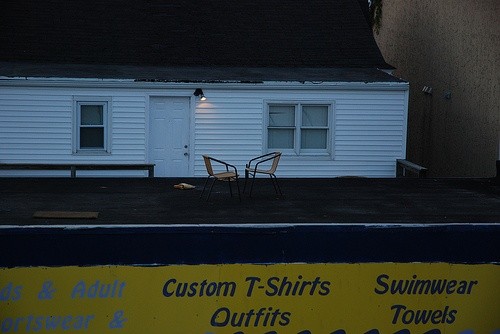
[0,162,155,185]
[396,157,428,179]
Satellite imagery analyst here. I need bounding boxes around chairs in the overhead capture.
[200,154,242,202]
[241,151,286,201]
